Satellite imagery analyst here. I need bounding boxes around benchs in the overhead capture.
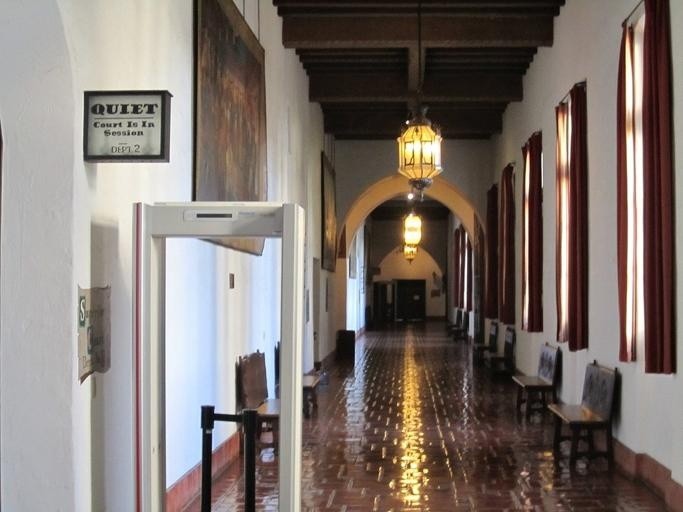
[445,310,619,478]
[235,350,278,458]
[276,342,319,419]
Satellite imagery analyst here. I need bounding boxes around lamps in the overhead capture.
[396,0,443,264]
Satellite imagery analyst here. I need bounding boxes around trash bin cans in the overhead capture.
[336,330,355,357]
